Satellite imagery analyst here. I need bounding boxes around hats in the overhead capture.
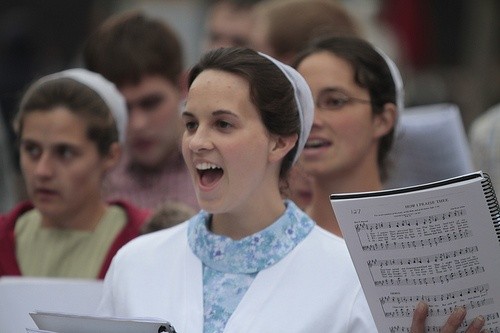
[257,51,314,168]
[15,68,128,153]
[373,47,403,137]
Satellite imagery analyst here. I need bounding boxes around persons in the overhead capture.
[83,12,202,216]
[290,38,405,237]
[409,300,487,333]
[0,68,195,281]
[98,47,378,333]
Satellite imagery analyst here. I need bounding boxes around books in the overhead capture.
[330,171,500,333]
